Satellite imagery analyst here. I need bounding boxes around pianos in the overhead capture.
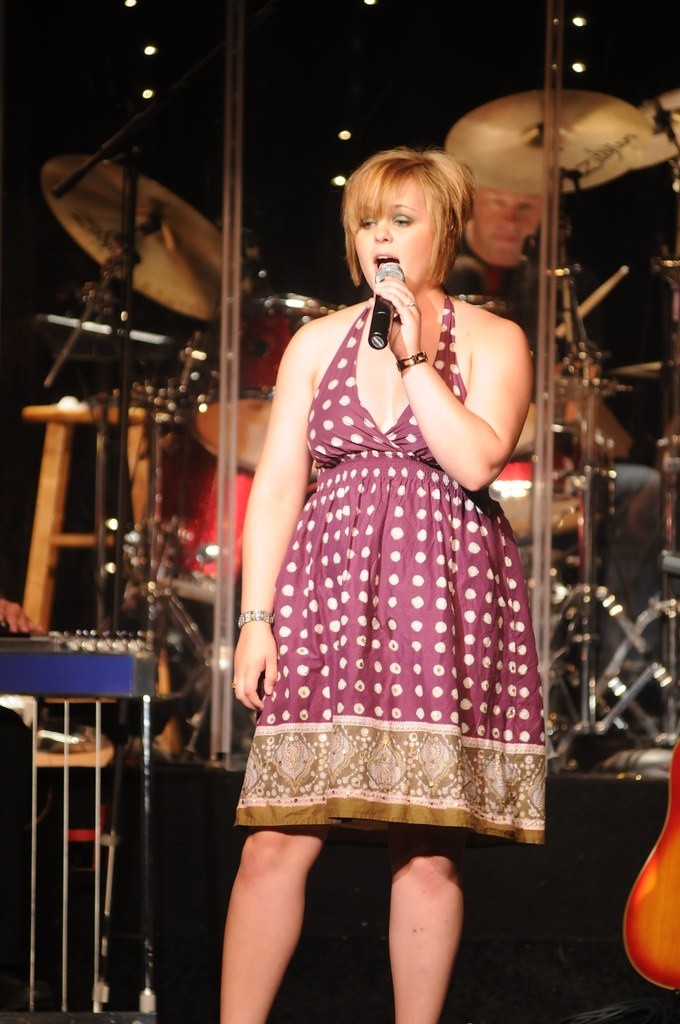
[0,625,170,703]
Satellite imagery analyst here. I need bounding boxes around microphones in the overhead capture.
[368,262,405,350]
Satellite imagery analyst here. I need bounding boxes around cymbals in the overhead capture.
[41,154,224,321]
[637,90,679,178]
[443,89,644,195]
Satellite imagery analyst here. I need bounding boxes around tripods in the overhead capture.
[91,417,223,703]
[546,169,680,774]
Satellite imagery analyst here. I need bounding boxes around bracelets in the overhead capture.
[237,610,275,628]
[396,351,429,371]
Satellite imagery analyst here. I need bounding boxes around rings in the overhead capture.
[407,301,415,307]
[232,683,236,688]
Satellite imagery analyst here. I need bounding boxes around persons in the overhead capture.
[441,185,666,752]
[219,149,548,1024]
[0,595,56,1010]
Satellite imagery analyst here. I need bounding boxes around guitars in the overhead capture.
[623,732,679,987]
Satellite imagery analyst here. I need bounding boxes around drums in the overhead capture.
[149,444,252,603]
[185,291,336,475]
[486,419,616,545]
